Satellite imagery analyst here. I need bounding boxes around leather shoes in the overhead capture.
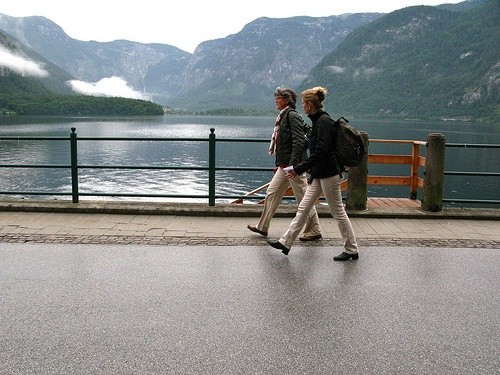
[299,234,322,241]
[333,251,358,261]
[247,224,268,236]
[266,240,290,256]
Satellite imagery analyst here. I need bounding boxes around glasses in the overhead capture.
[274,97,285,101]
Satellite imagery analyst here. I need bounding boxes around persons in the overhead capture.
[266,87,359,261]
[247,86,323,242]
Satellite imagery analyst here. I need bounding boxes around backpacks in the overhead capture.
[334,120,369,166]
